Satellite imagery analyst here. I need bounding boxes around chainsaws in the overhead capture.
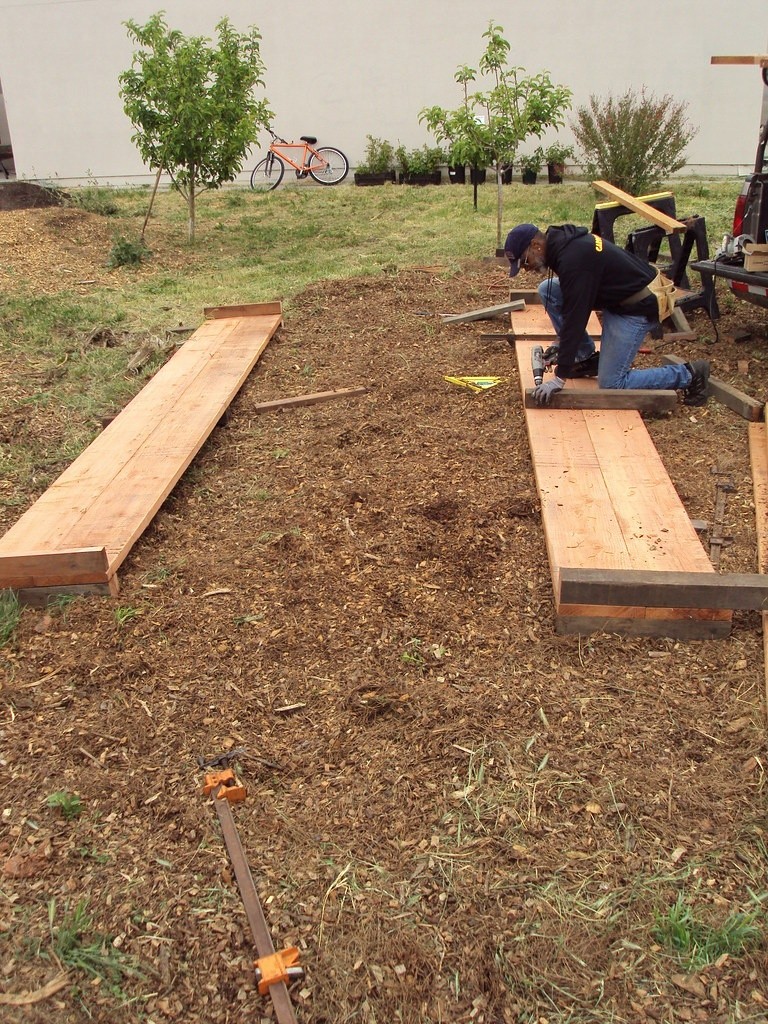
[715,231,756,264]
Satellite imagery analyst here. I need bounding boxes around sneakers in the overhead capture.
[682,359,710,407]
[553,351,600,378]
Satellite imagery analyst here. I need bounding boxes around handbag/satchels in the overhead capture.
[619,264,676,323]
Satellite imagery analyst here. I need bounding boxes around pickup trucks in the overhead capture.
[687,173,768,306]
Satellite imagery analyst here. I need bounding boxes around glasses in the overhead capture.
[520,244,531,269]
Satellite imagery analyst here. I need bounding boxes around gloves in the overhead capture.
[542,346,560,373]
[530,376,566,408]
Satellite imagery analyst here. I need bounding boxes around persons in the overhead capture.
[504,223,710,407]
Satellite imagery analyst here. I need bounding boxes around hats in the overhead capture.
[504,223,538,277]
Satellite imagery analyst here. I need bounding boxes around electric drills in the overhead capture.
[530,345,560,388]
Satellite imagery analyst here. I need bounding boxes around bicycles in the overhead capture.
[250,128,348,188]
